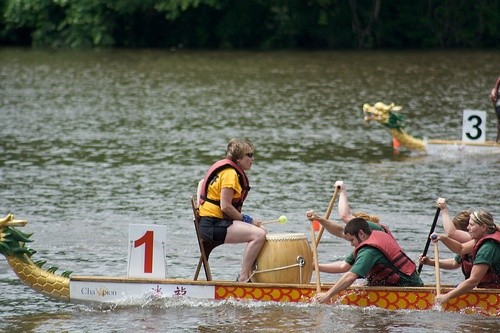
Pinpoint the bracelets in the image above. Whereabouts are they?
[241,213,253,224]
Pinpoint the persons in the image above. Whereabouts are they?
[197,138,267,284]
[306,181,424,304]
[430,210,500,306]
[491,76,500,143]
[419,197,474,280]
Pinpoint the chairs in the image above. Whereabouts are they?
[190,195,222,281]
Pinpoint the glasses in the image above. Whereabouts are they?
[243,152,255,157]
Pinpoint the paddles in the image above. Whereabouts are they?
[417,208,441,273]
[431,235,444,311]
[308,212,322,293]
[312,186,341,253]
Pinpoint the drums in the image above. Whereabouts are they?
[249,232,313,284]
